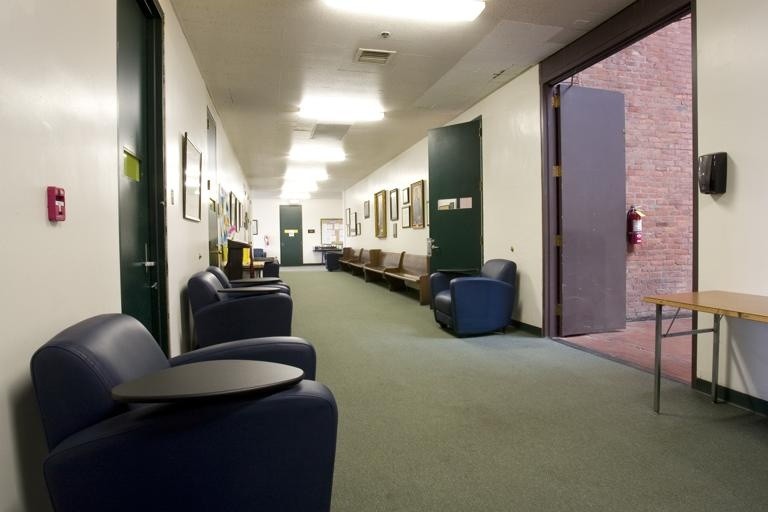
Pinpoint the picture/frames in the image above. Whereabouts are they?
[181,132,259,236]
[320,180,429,245]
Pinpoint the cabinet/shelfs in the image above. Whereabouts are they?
[227,239,254,279]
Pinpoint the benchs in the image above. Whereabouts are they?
[337,247,432,306]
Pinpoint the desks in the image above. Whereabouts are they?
[641,289,768,414]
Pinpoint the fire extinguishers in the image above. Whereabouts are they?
[627,205,646,244]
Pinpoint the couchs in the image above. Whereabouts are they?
[205,265,292,289]
[28,314,339,511]
[187,271,293,348]
[431,258,517,338]
